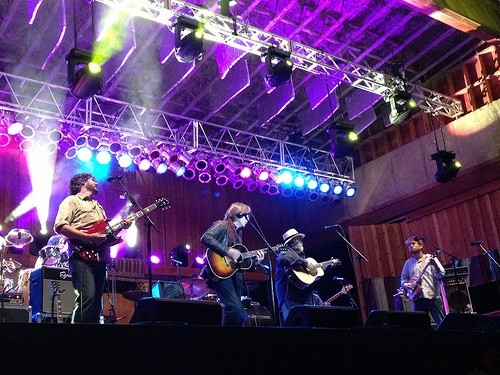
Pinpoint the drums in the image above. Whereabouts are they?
[38,245,61,265]
[18,268,37,305]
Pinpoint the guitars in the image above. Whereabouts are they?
[66,198,172,261]
[203,244,289,282]
[285,257,341,290]
[50,281,63,323]
[403,248,441,301]
[320,283,353,306]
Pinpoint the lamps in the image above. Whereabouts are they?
[427,111,462,184]
[169,244,191,267]
[388,90,422,126]
[10,242,26,255]
[0,109,357,205]
[325,79,358,158]
[170,16,205,64]
[64,0,105,100]
[30,227,52,257]
[260,45,294,88]
[192,255,205,268]
[123,240,140,260]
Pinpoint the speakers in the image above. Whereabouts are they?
[29,265,78,315]
[438,312,500,331]
[362,310,431,330]
[130,298,222,326]
[284,304,364,328]
[444,274,474,313]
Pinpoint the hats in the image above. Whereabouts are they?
[282,228,305,247]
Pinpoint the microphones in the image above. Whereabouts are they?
[333,277,344,281]
[236,212,250,218]
[414,236,426,242]
[324,225,340,231]
[470,240,483,246]
[107,176,124,182]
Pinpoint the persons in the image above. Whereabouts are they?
[53,172,132,324]
[200,202,265,327]
[275,228,338,321]
[399,236,449,326]
